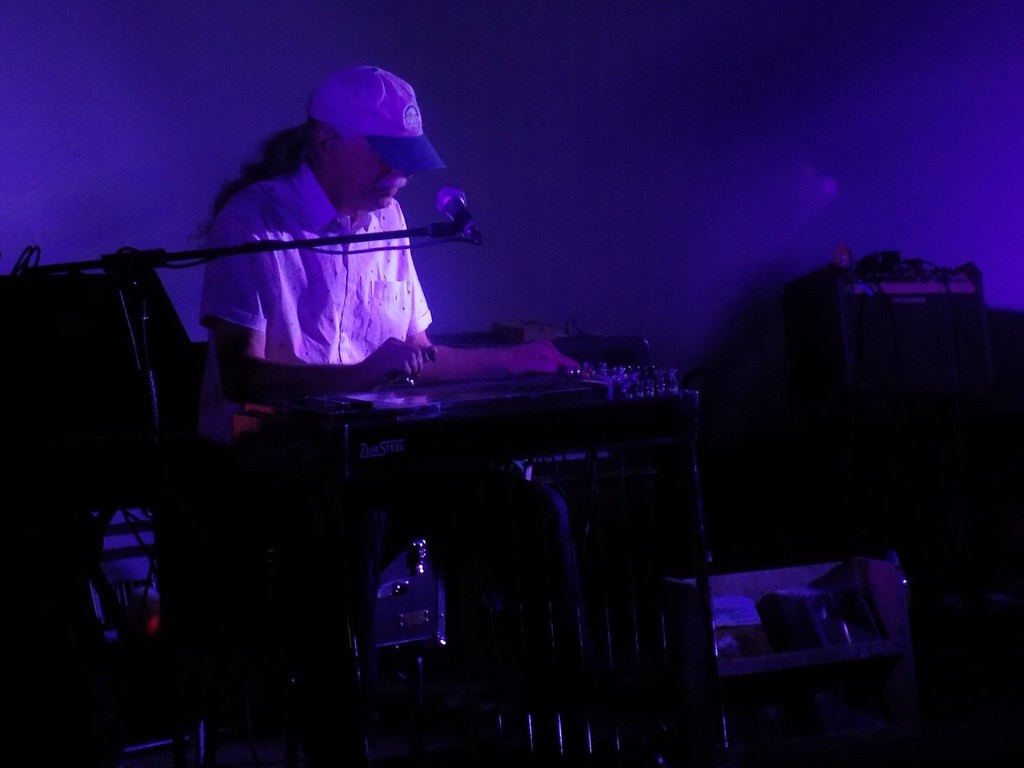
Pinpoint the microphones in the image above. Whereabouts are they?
[436,186,484,244]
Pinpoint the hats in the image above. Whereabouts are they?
[309,66,446,174]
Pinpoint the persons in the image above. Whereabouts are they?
[198,65,585,671]
